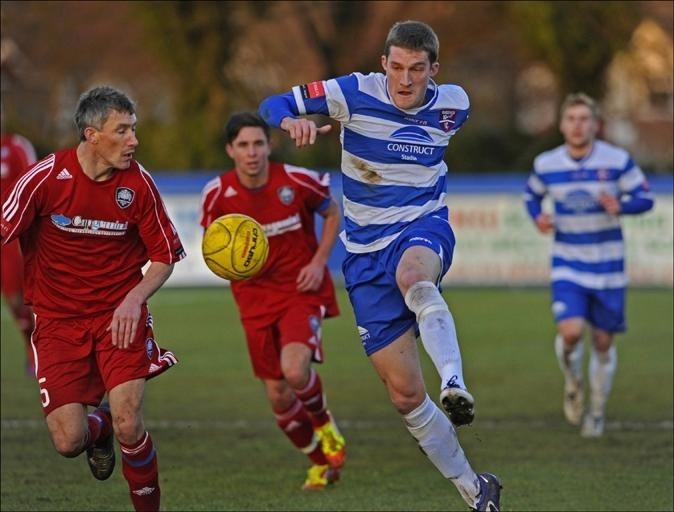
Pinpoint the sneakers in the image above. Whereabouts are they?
[86,403,115,479]
[440,375,475,426]
[468,473,503,511]
[301,463,341,490]
[564,373,605,438]
[307,407,347,468]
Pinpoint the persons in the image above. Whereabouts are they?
[520,93,659,443]
[258,16,502,511]
[0,82,177,512]
[0,130,39,379]
[196,111,350,491]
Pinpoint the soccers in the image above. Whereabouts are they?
[202,214,271,280]
